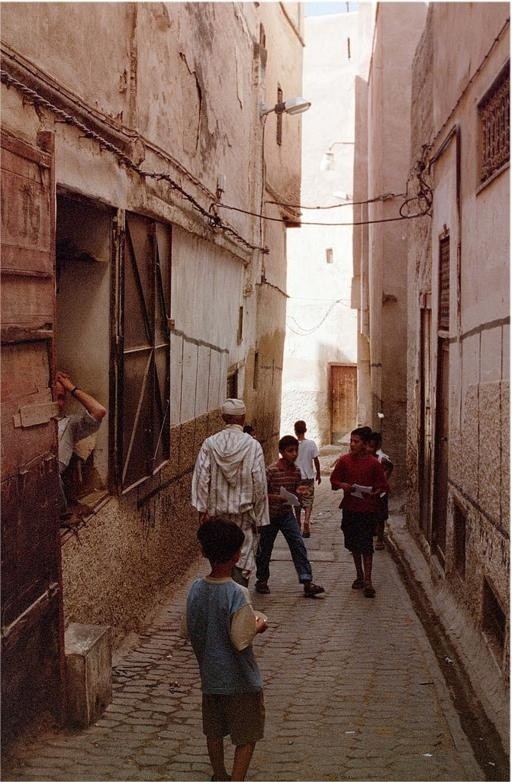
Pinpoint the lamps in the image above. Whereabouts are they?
[260,97,312,118]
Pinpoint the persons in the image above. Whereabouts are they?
[56,371,108,523]
[329,426,393,597]
[244,424,257,440]
[253,436,325,598]
[293,421,321,538]
[189,397,270,590]
[177,517,269,781]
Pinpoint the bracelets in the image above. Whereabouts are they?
[70,386,81,395]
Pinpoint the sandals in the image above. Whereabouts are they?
[352,580,375,598]
[255,581,270,594]
[304,583,324,598]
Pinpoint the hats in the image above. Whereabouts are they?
[221,398,246,416]
